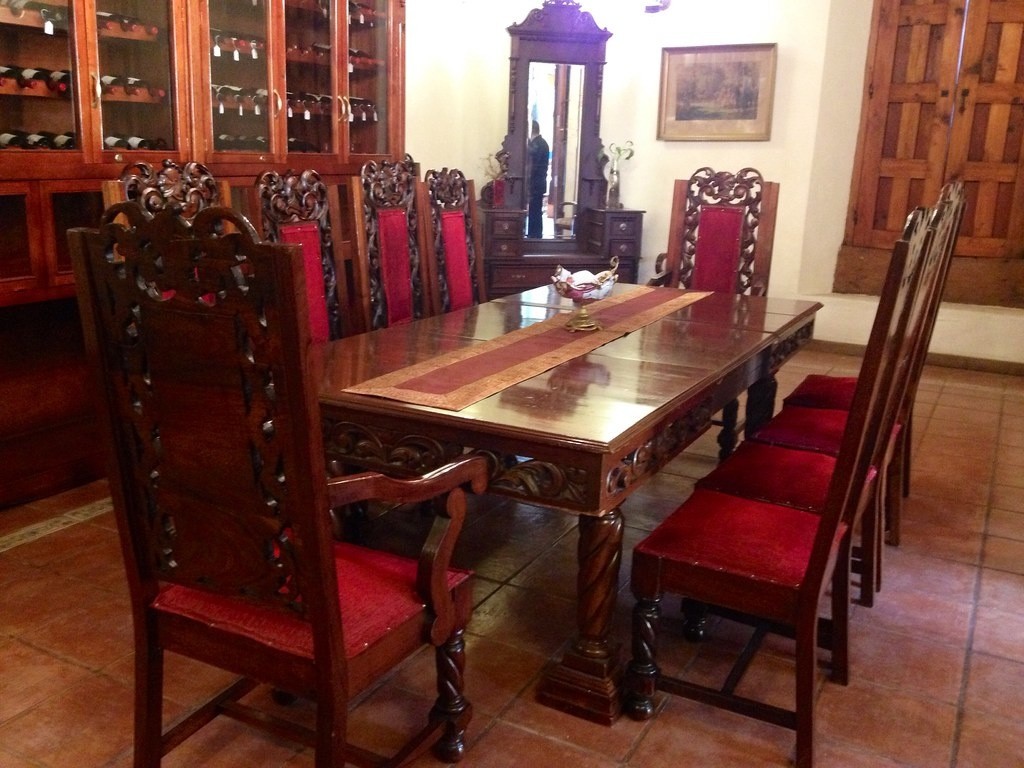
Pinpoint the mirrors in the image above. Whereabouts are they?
[523,59,586,242]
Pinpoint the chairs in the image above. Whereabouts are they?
[65,154,483,768]
[643,168,777,459]
[753,170,966,546]
[695,200,956,607]
[627,201,932,768]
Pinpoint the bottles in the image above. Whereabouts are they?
[317,0,379,19]
[0,130,154,151]
[210,25,386,69]
[0,0,159,36]
[0,62,168,98]
[211,81,379,114]
[215,132,319,153]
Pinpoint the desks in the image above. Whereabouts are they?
[265,283,824,727]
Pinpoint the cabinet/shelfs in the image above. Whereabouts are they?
[476,0,646,303]
[0,0,405,508]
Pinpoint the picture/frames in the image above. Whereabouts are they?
[655,42,777,141]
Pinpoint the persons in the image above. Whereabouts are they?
[527,120,550,239]
